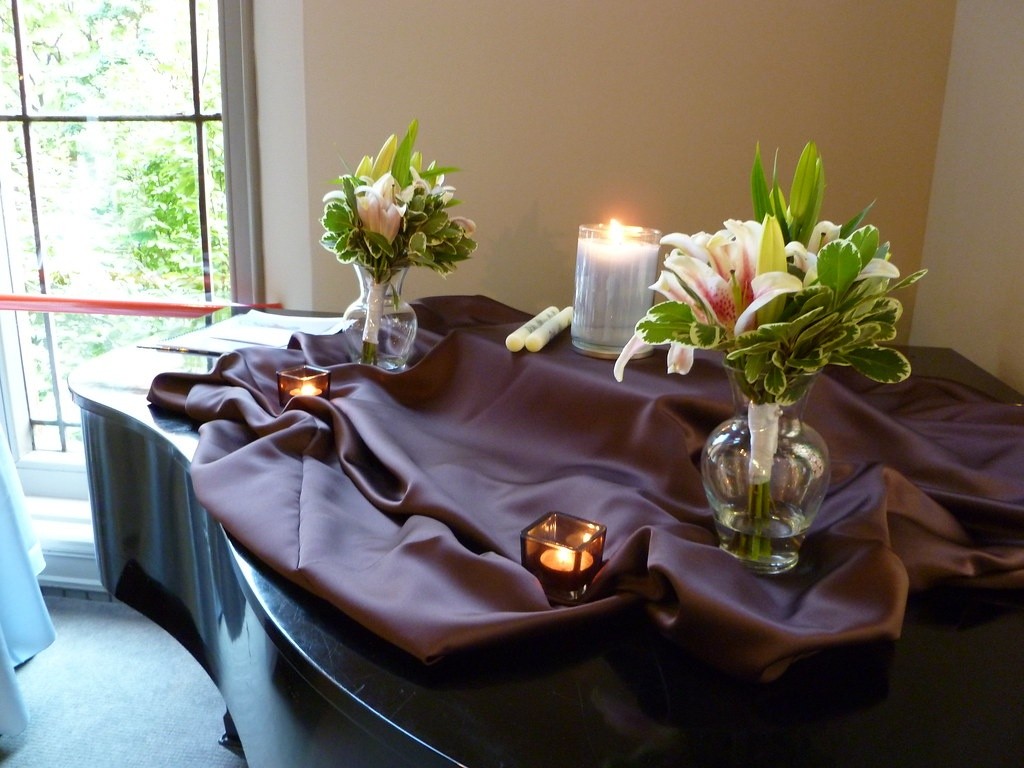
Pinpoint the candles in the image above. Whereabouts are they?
[277,364,331,408]
[572,218,660,356]
[519,512,605,603]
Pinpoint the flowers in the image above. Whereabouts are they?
[617,136,923,402]
[315,115,476,277]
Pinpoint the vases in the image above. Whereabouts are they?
[340,262,417,373]
[701,361,829,580]
